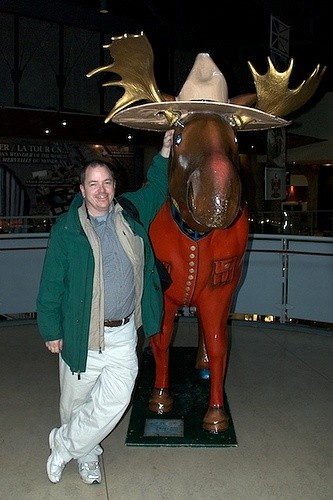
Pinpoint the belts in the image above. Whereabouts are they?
[104,311,133,327]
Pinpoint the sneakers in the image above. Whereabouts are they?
[78,461,102,485]
[46,427,67,483]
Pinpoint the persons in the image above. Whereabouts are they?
[35,129,174,485]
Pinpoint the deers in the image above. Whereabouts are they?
[86,30,327,442]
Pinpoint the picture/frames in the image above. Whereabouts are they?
[265,167,286,201]
